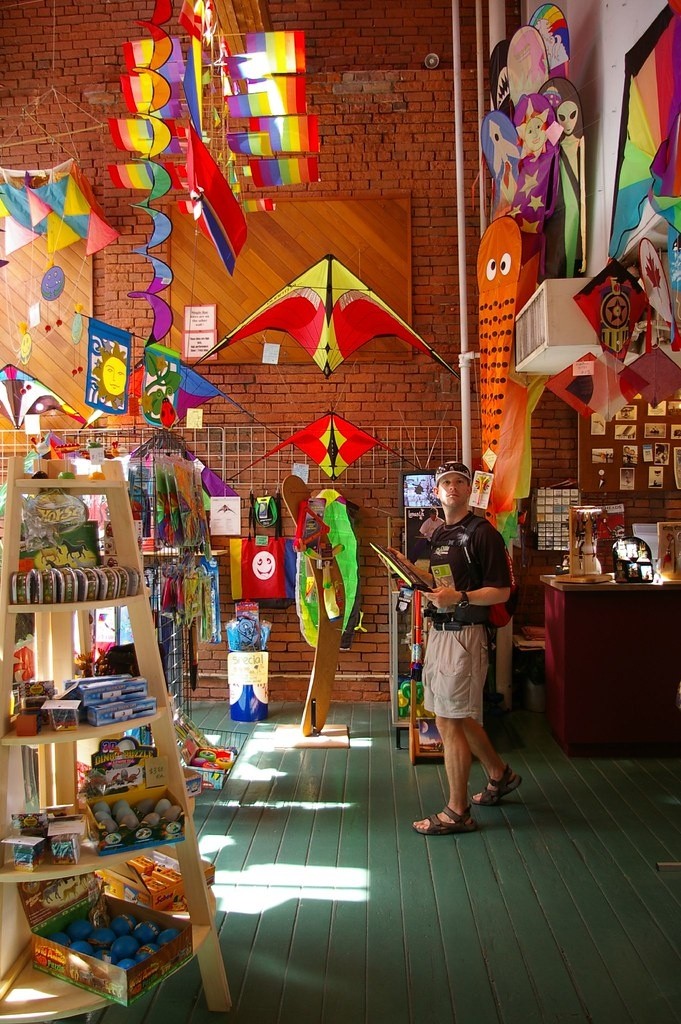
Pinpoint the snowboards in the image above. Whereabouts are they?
[281,474,346,737]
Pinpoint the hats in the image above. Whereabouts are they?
[435,461,472,487]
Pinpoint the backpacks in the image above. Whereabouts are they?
[254,496,277,528]
[430,517,517,627]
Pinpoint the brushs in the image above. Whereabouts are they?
[412,590,423,682]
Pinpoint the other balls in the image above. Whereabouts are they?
[48,913,179,970]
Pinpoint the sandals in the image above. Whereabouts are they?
[412,803,477,834]
[471,763,522,805]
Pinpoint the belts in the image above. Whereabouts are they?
[433,621,481,631]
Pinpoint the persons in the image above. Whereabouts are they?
[417,508,445,558]
[389,461,524,836]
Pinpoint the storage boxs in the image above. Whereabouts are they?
[63,674,132,691]
[17,873,194,1008]
[77,676,149,707]
[85,788,186,855]
[86,695,158,727]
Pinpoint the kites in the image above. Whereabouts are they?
[0,0,681,656]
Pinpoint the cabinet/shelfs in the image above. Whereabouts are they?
[0,458,232,1024]
[410,593,444,765]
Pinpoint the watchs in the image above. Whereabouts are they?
[458,591,469,608]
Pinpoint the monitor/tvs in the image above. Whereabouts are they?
[399,469,442,518]
